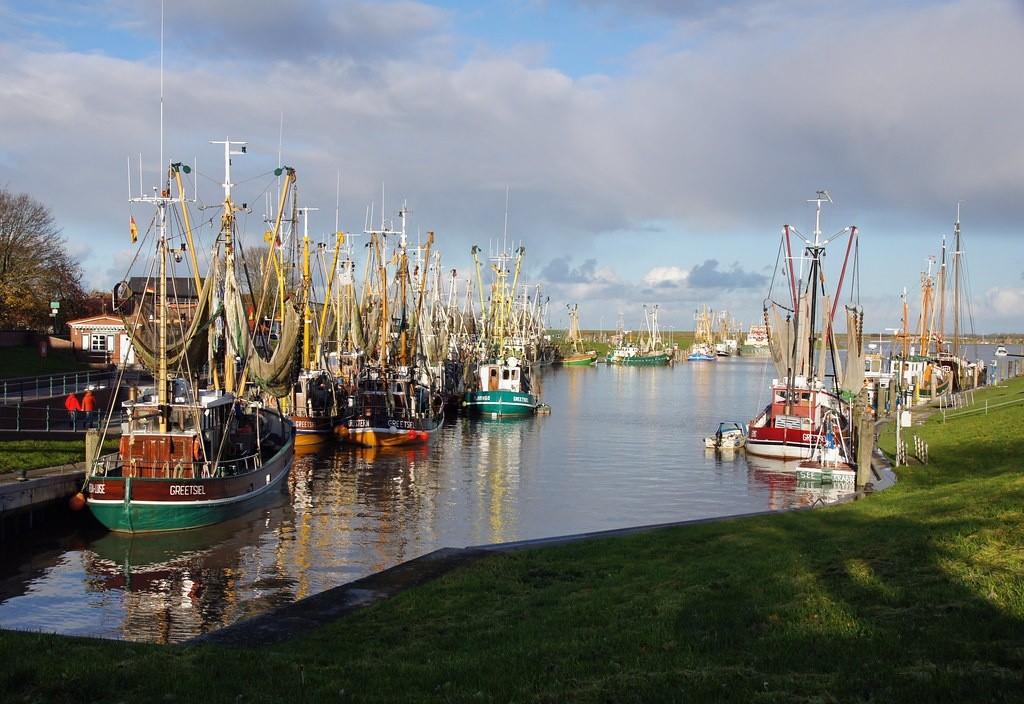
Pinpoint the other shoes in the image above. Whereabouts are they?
[89,426,95,428]
[83,423,86,428]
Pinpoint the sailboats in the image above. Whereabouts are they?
[69,157,1010,536]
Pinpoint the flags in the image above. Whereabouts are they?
[129,215,138,244]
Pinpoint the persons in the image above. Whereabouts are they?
[65,392,82,430]
[82,390,97,428]
